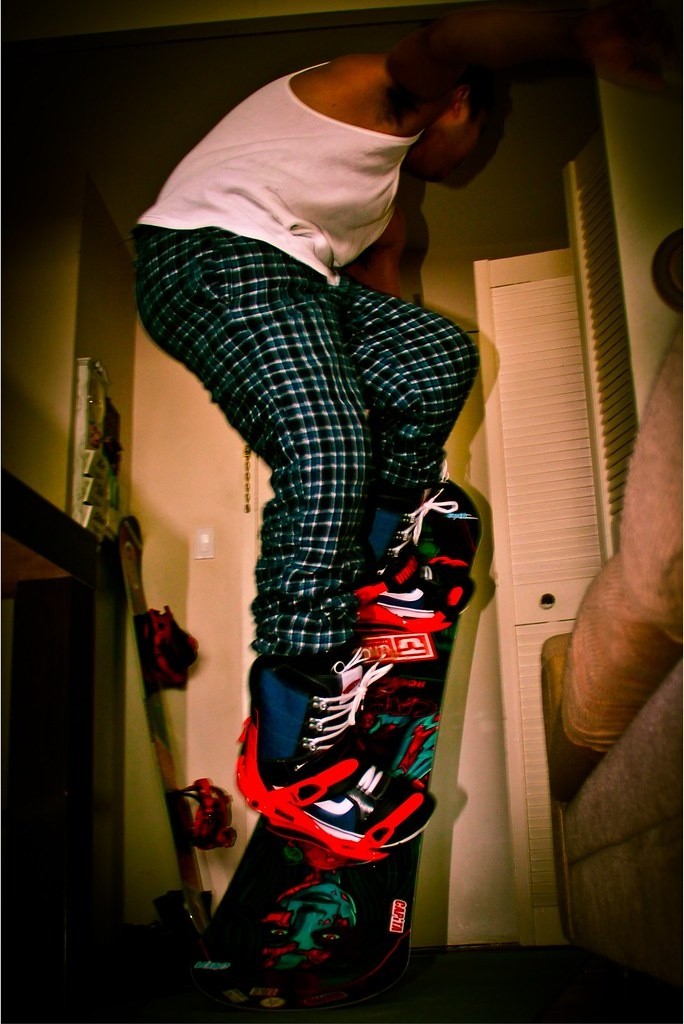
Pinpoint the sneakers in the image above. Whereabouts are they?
[235,759,437,850]
[355,561,476,620]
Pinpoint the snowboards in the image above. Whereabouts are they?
[117,515,237,935]
[193,481,483,1012]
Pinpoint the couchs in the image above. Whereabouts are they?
[541,325,683,987]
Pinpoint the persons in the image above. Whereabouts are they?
[133,0,682,854]
